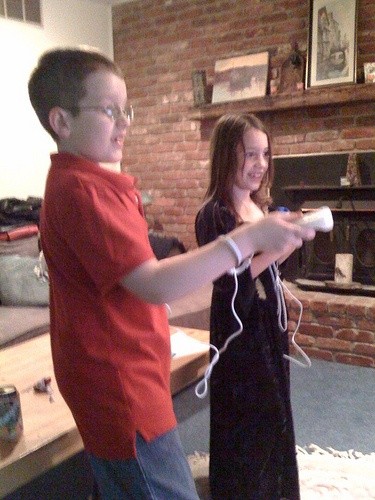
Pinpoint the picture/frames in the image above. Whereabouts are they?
[303,0,361,91]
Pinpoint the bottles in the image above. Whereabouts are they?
[1,384,22,445]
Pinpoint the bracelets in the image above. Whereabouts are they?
[216,232,244,267]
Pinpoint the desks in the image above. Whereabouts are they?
[0,325,209,500]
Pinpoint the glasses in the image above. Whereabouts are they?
[76,103,135,120]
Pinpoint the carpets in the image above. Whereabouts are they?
[185,442,375,500]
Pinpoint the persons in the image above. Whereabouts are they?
[27,47,315,500]
[194,110,304,500]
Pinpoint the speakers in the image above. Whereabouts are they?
[350,214,375,283]
[303,215,349,281]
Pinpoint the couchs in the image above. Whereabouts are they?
[0,224,186,348]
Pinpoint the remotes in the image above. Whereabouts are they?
[290,208,334,237]
